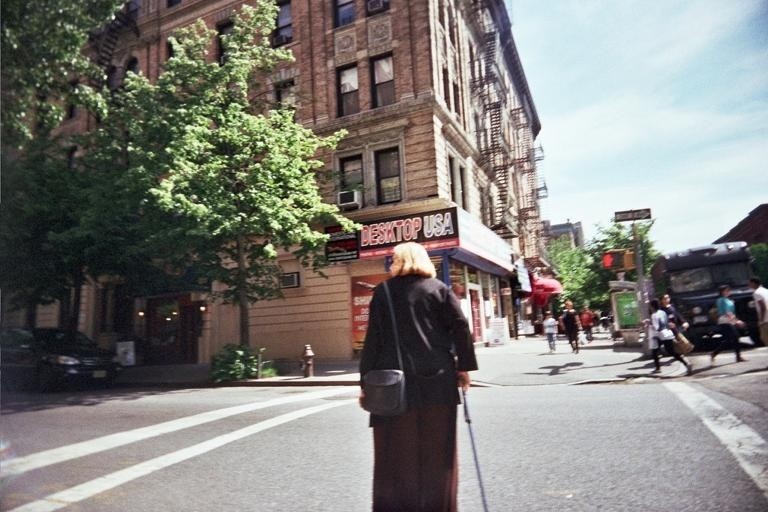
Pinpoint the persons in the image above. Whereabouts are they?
[662,293,689,337]
[559,300,582,353]
[641,298,693,374]
[356,240,480,512]
[581,306,594,339]
[543,310,558,351]
[710,284,747,361]
[746,276,768,346]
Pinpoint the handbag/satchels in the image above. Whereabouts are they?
[362,368,408,416]
[672,331,696,354]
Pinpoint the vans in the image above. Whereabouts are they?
[650,241,763,353]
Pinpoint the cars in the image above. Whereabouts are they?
[0,326,117,393]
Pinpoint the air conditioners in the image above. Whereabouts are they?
[337,190,363,212]
